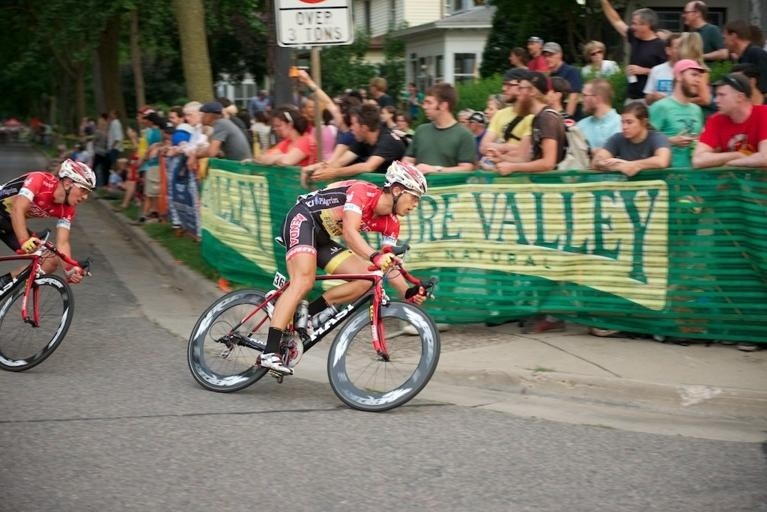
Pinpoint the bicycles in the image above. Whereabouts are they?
[0,225,97,373]
[183,234,444,416]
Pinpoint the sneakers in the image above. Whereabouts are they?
[522,318,567,334]
[31,228,51,246]
[256,350,293,375]
[433,313,454,332]
[0,273,14,295]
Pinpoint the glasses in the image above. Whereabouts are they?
[589,51,601,56]
[579,92,595,99]
[682,10,697,14]
[501,82,518,86]
[517,85,531,92]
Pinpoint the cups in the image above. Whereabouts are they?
[624,74,638,84]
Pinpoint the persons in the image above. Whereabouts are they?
[199,70,479,335]
[592,0,767,351]
[457,38,623,333]
[5,117,53,145]
[57,96,250,239]
[254,159,435,375]
[0,157,96,293]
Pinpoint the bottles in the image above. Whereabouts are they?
[293,299,309,331]
[310,304,339,330]
[687,132,699,158]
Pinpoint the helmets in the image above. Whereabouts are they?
[383,159,427,199]
[58,159,97,192]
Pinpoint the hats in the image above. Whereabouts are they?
[521,72,548,95]
[673,58,711,79]
[540,42,562,56]
[136,106,154,115]
[143,113,158,121]
[711,73,744,93]
[465,113,484,124]
[198,101,223,113]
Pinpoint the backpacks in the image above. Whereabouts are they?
[544,108,590,183]
